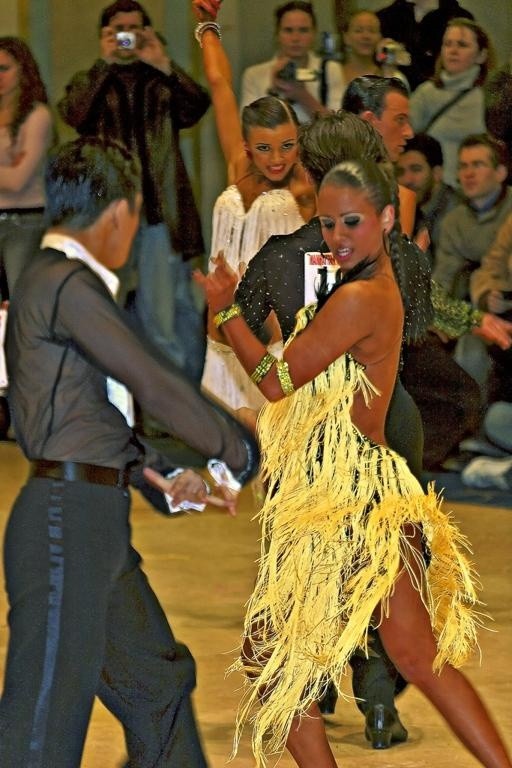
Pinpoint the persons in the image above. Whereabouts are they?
[1,37,54,302]
[336,75,512,471]
[191,2,317,501]
[469,214,512,489]
[194,163,512,768]
[402,19,504,208]
[337,11,412,100]
[393,133,465,273]
[224,112,432,749]
[59,1,209,381]
[239,1,345,132]
[430,136,511,448]
[0,138,254,768]
[374,1,476,98]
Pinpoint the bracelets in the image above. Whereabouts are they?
[212,303,243,330]
[273,360,296,398]
[194,20,224,45]
[248,352,275,388]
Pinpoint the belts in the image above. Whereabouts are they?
[31,457,130,487]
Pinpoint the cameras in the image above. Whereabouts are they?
[109,29,139,53]
[273,62,318,85]
[375,41,408,67]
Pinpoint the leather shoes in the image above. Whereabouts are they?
[365,705,407,750]
[318,680,339,714]
